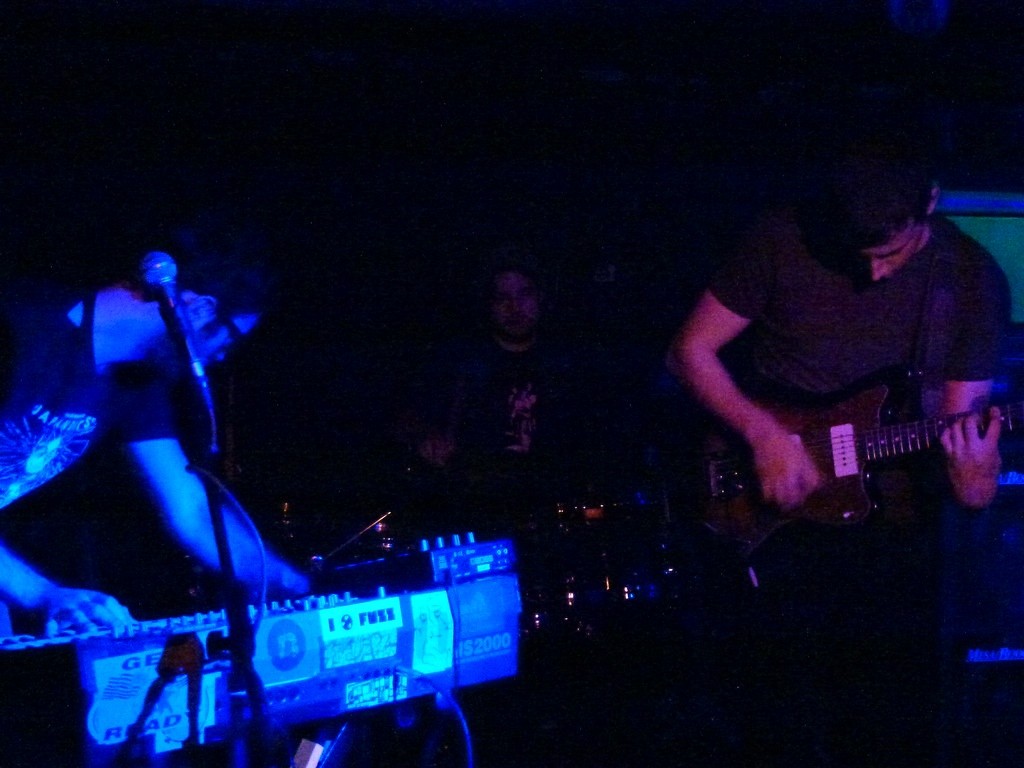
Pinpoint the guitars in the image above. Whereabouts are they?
[701,385,1024,527]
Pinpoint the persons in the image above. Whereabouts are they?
[390,231,637,768]
[660,104,1015,768]
[0,196,312,766]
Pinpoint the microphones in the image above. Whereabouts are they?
[141,251,215,423]
